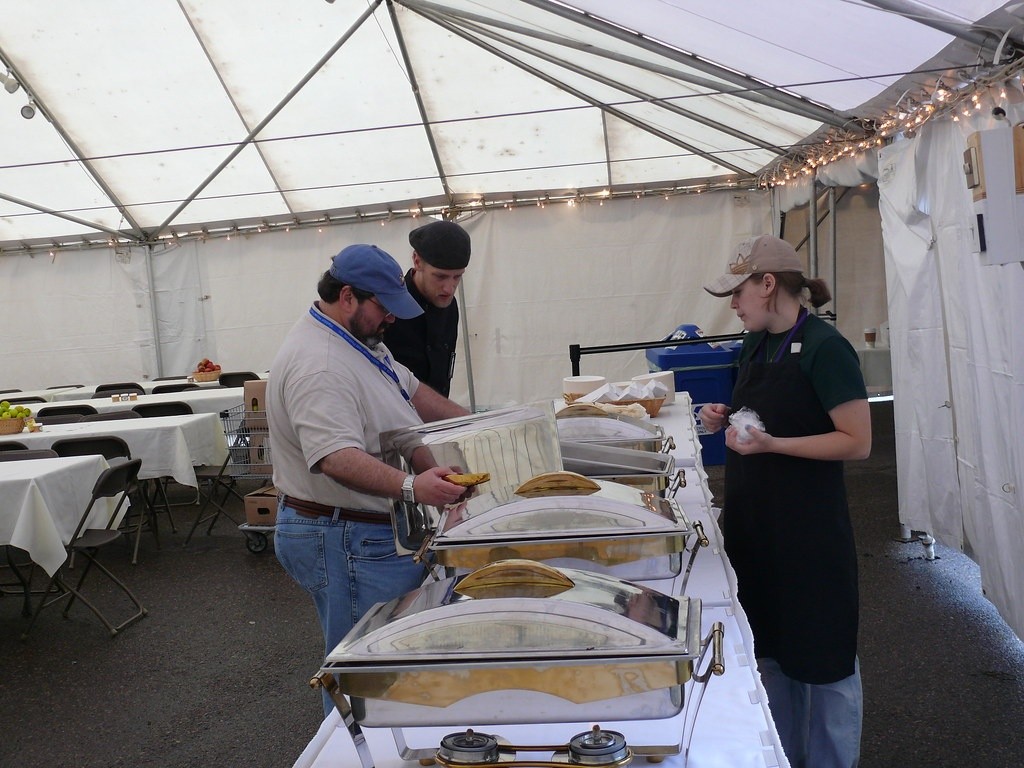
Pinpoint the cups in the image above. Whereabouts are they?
[864,328,876,348]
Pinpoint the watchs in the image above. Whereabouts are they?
[400,474,417,507]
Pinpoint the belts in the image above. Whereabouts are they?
[277,493,391,525]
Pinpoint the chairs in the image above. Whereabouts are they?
[20,458,149,643]
[133,401,220,509]
[0,442,28,451]
[52,435,160,566]
[34,414,84,425]
[92,389,144,399]
[77,410,143,423]
[219,372,260,387]
[182,419,250,546]
[96,382,146,395]
[152,384,200,394]
[0,397,48,403]
[37,405,98,416]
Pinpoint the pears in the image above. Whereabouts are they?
[0,401,30,418]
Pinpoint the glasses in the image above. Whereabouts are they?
[350,289,391,318]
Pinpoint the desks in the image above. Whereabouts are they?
[0,413,233,547]
[291,391,792,768]
[0,454,104,618]
[10,386,248,458]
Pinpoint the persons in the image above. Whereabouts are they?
[381,221,472,399]
[697,233,873,768]
[265,244,474,719]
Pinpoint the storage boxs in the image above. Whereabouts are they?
[111,394,120,402]
[431,498,692,582]
[250,428,273,474]
[589,426,667,452]
[244,380,268,430]
[121,394,128,401]
[129,393,137,400]
[244,486,278,526]
[323,597,703,728]
[589,458,676,498]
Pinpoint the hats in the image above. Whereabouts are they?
[704,233,804,297]
[409,221,471,270]
[328,244,425,320]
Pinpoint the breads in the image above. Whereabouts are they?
[444,470,490,485]
